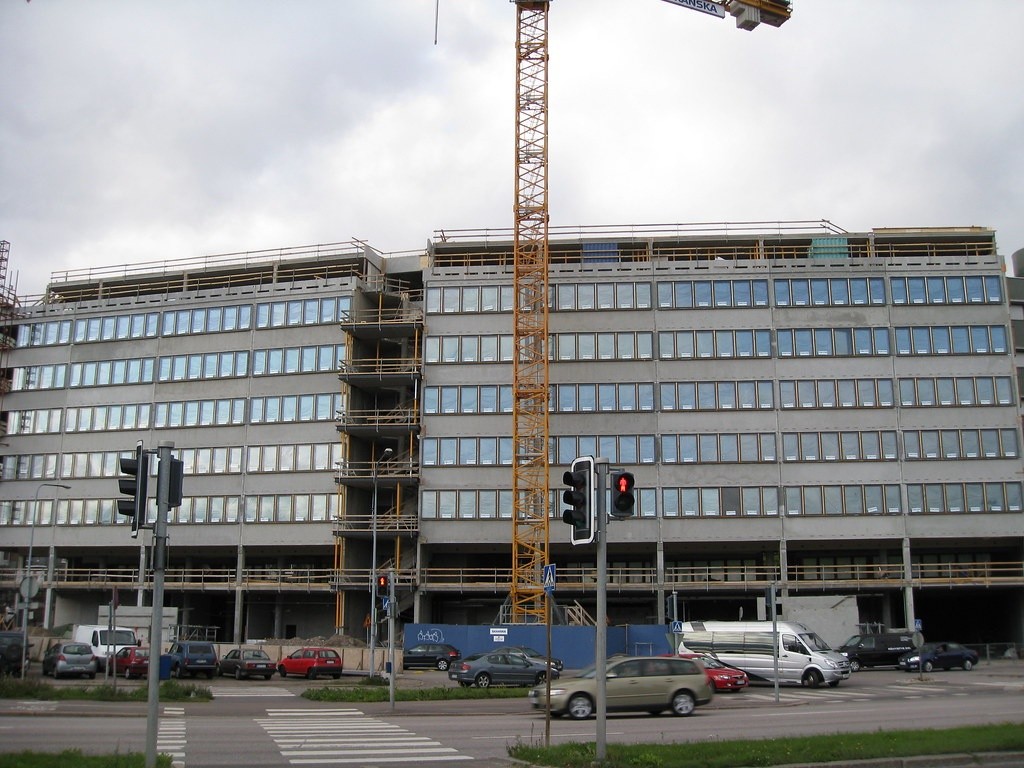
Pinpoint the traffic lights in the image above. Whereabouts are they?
[377,574,388,598]
[117,440,148,539]
[610,472,635,518]
[562,455,595,545]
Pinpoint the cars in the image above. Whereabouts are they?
[657,654,750,692]
[528,656,714,720]
[898,642,979,672]
[42,642,98,679]
[448,647,564,688]
[278,648,342,680]
[220,649,276,680]
[404,643,461,671]
[108,647,151,679]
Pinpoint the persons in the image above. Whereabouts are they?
[618,665,633,676]
[937,646,945,653]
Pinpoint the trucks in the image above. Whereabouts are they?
[75,625,141,673]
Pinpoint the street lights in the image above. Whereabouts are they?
[370,448,393,674]
[22,484,72,681]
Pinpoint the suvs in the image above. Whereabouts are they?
[165,642,219,679]
[0,632,35,677]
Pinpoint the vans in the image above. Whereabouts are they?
[839,634,918,672]
[678,621,851,688]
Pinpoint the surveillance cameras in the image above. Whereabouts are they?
[385,448,393,456]
